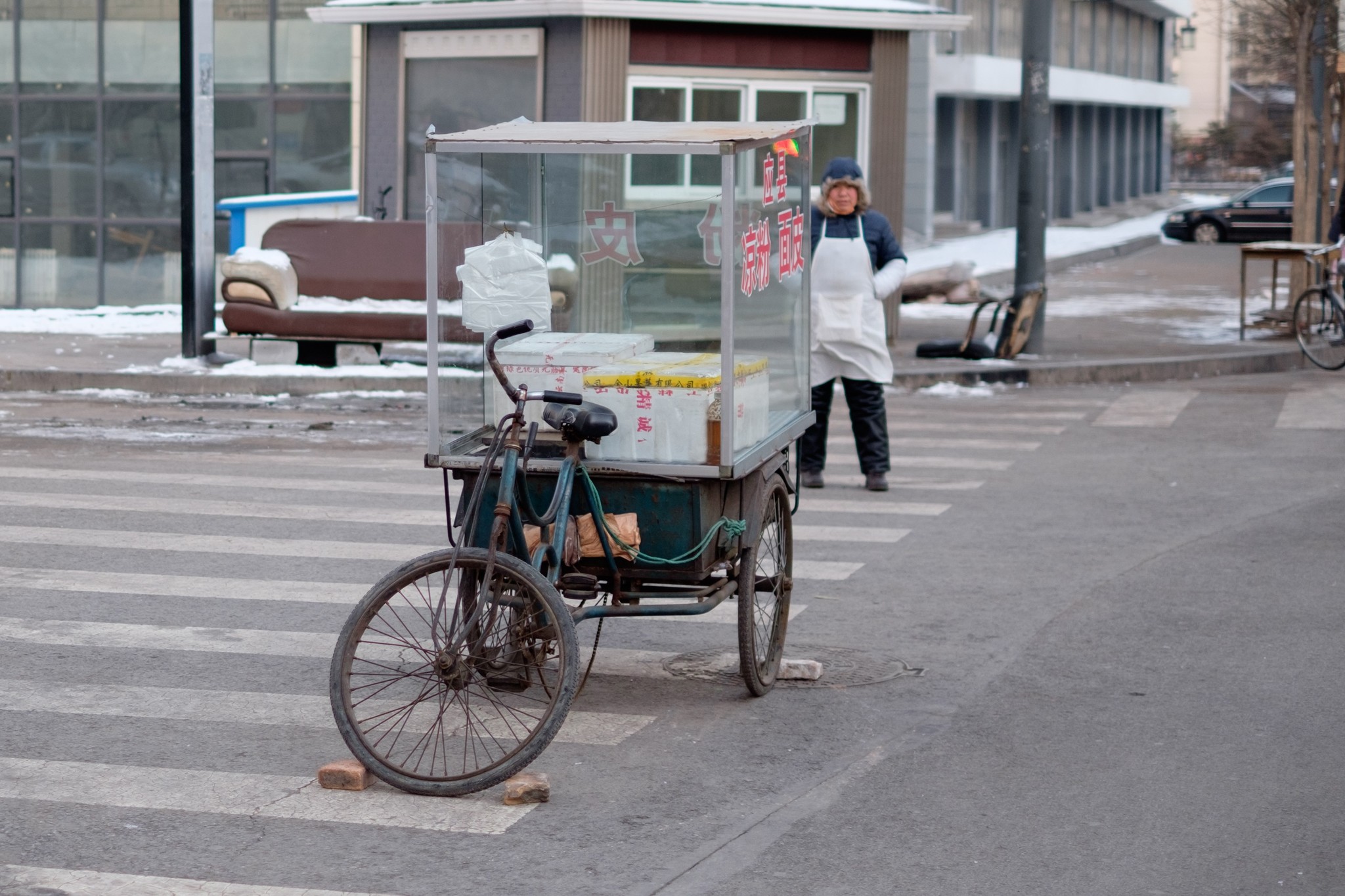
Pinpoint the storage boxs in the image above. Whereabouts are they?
[493,331,655,431]
[579,352,769,466]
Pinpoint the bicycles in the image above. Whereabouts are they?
[1291,235,1345,371]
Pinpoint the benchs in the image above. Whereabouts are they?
[221,217,579,368]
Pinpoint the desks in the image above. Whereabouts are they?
[1239,242,1335,343]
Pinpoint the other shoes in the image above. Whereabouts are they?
[800,471,824,488]
[865,472,889,492]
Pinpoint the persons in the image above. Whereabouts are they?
[796,156,908,490]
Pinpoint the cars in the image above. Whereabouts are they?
[1159,175,1345,245]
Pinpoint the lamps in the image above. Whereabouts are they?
[1180,18,1196,50]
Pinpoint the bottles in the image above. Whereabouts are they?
[706,391,721,467]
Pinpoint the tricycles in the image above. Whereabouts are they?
[327,317,795,799]
